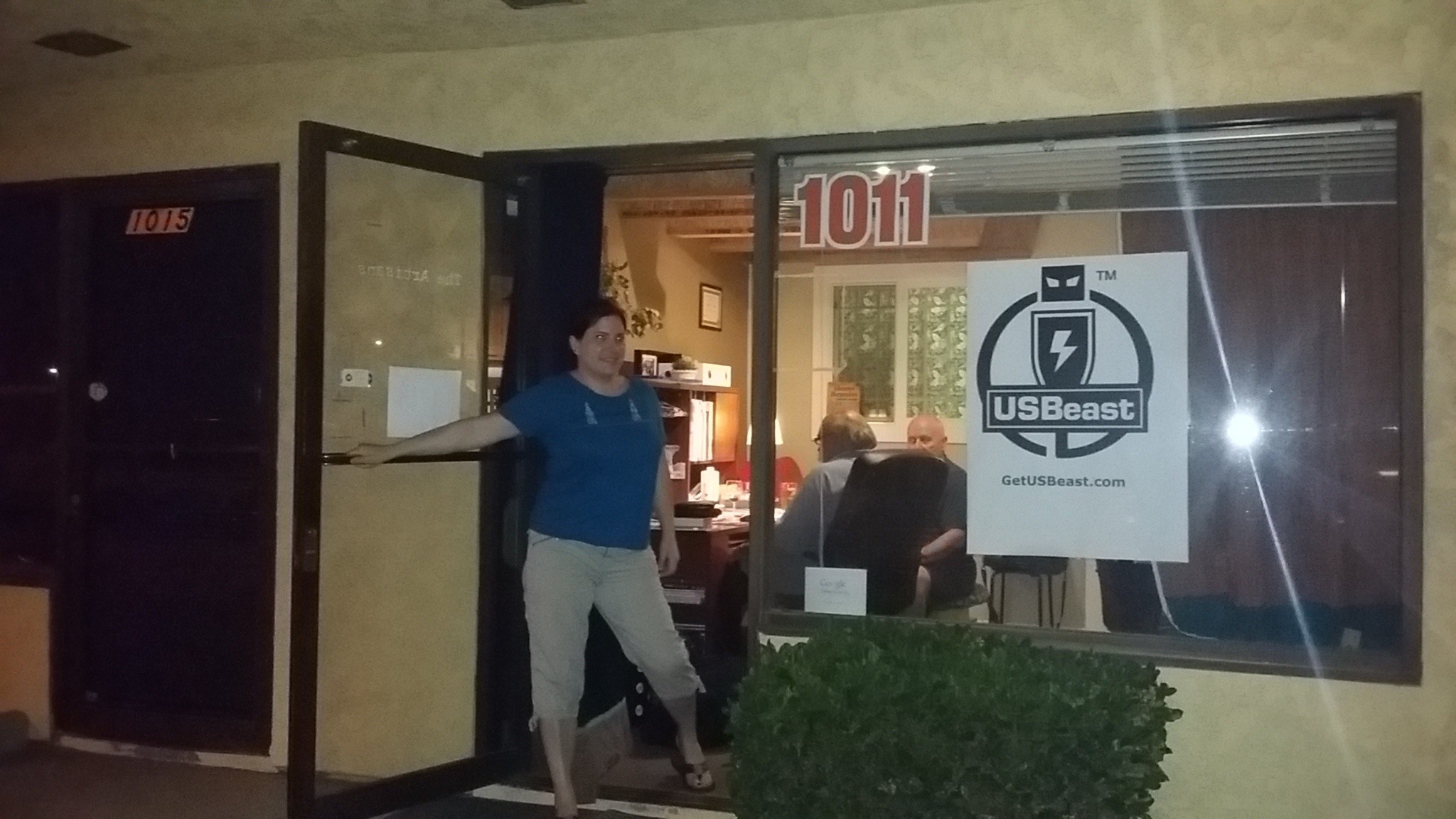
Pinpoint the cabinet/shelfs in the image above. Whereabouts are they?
[638,378,739,505]
[644,507,795,749]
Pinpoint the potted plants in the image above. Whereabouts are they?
[672,357,698,381]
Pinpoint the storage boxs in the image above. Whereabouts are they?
[697,363,732,388]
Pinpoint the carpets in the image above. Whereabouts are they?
[386,796,662,819]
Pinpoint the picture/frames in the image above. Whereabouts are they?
[700,282,724,332]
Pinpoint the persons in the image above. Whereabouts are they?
[906,415,977,618]
[703,410,878,747]
[344,294,715,819]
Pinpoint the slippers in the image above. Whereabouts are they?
[671,748,715,792]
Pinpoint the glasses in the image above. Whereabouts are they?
[814,434,828,445]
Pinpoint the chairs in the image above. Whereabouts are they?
[736,456,804,499]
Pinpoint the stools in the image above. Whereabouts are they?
[981,556,1068,628]
[925,582,990,621]
